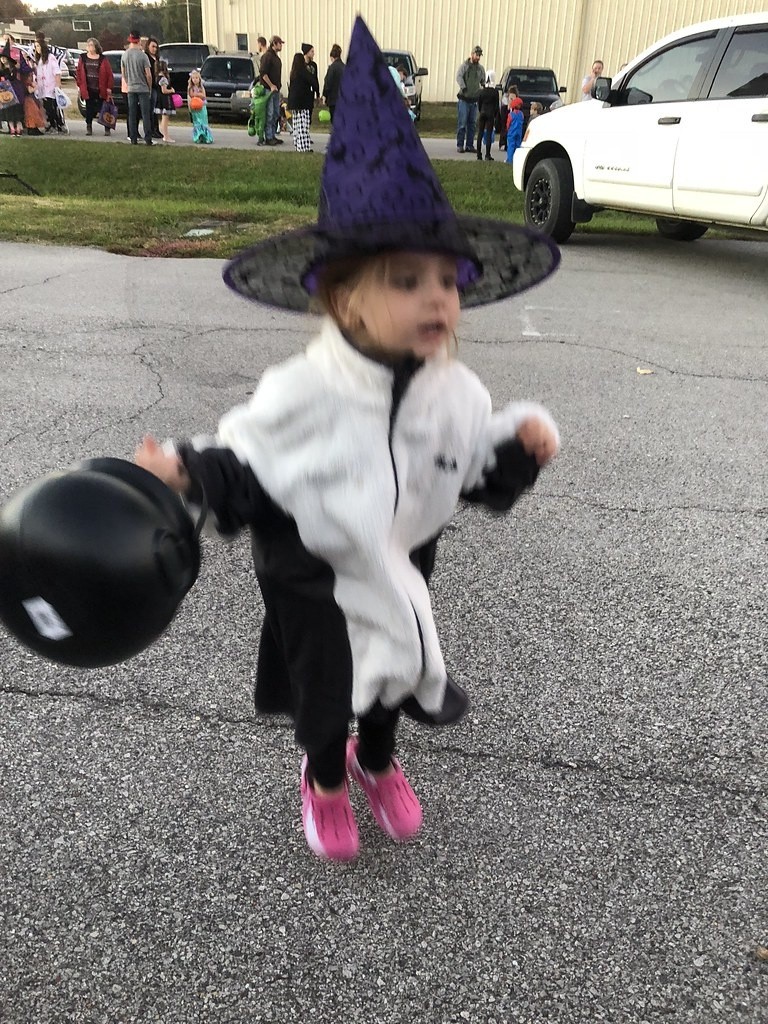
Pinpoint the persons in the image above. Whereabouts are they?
[0,26,69,137]
[497,85,542,163]
[456,46,486,154]
[76,37,115,136]
[288,43,320,153]
[187,69,213,144]
[323,44,346,124]
[582,60,604,101]
[250,36,284,153]
[476,70,501,160]
[134,247,562,863]
[119,32,176,143]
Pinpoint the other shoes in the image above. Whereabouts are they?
[163,138,176,144]
[485,155,495,161]
[274,138,283,144]
[476,153,482,160]
[465,146,477,152]
[266,137,276,146]
[85,125,92,135]
[104,126,110,136]
[457,146,464,153]
[28,128,43,136]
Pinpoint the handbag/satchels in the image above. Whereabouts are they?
[98,98,118,130]
[0,79,20,109]
[56,87,72,108]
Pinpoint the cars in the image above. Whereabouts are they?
[0,40,85,82]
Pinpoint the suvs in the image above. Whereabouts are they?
[198,51,262,119]
[156,42,217,101]
[379,48,429,123]
[495,65,568,134]
[75,49,140,118]
[511,10,768,246]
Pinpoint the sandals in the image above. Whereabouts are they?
[348,740,424,839]
[301,754,359,860]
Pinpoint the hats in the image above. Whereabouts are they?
[0,38,17,65]
[269,35,285,43]
[16,51,33,73]
[127,30,140,42]
[301,43,313,53]
[472,46,483,56]
[330,44,342,57]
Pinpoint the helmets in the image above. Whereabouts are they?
[223,16,562,315]
